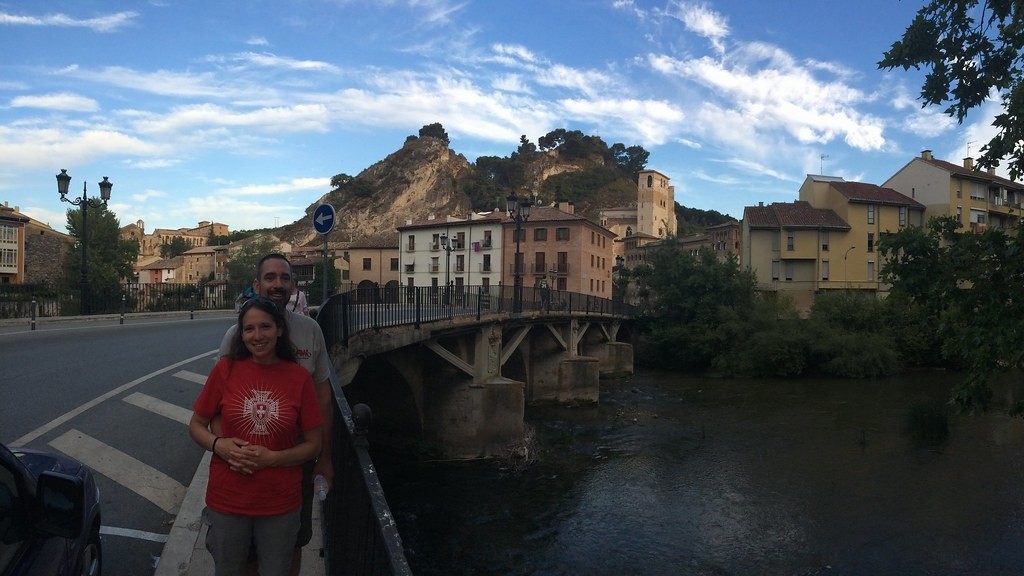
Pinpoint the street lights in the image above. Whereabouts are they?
[504,191,532,314]
[55,168,113,316]
[439,232,458,305]
[615,255,625,315]
[548,268,558,308]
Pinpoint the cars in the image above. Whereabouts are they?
[0,440,105,576]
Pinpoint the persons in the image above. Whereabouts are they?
[241,286,259,301]
[286,273,310,317]
[538,275,551,310]
[189,299,323,576]
[222,254,333,576]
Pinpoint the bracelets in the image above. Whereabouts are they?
[212,437,220,455]
